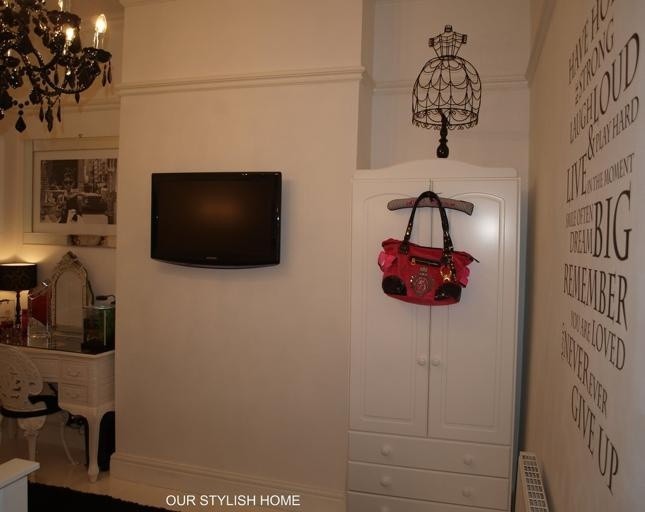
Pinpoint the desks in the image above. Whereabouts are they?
[0,337,115,483]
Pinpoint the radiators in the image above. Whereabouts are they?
[515,452,550,512]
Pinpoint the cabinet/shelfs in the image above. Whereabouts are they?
[352,159,523,512]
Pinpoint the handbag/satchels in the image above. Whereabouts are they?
[379,237,480,305]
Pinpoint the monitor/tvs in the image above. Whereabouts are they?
[148,170,283,271]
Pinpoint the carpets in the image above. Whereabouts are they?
[27,482,181,512]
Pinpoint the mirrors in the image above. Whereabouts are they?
[51,251,94,338]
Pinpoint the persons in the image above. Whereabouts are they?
[56,170,89,226]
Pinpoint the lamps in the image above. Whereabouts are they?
[0,0,113,131]
[0,262,38,328]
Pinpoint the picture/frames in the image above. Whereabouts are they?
[22,136,118,250]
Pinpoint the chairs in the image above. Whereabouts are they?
[0,347,77,484]
